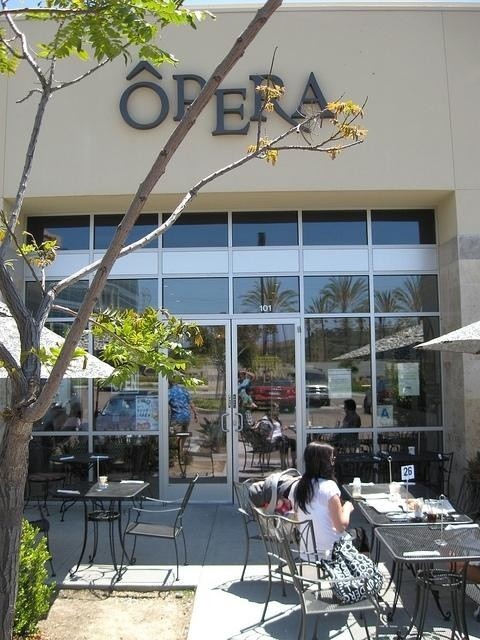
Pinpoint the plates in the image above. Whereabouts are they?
[386,511,427,522]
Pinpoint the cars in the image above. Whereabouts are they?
[99,383,120,393]
[362,373,398,416]
[247,374,297,411]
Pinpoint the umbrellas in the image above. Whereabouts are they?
[414,320,479,356]
[0,300,122,382]
[327,327,422,366]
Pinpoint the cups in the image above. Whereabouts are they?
[425,498,439,520]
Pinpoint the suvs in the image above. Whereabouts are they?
[71,391,181,478]
[288,365,333,407]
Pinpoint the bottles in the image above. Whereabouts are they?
[353,476,362,497]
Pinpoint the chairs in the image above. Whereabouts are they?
[233,480,296,583]
[22,413,220,580]
[276,525,392,640]
[241,409,427,477]
[248,504,337,626]
[233,443,480,521]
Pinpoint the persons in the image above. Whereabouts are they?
[447,537,480,623]
[166,372,200,465]
[233,367,297,470]
[283,441,374,565]
[34,396,85,456]
[330,397,363,448]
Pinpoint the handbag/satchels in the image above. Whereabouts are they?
[315,536,384,606]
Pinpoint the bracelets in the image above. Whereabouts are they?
[194,412,198,416]
